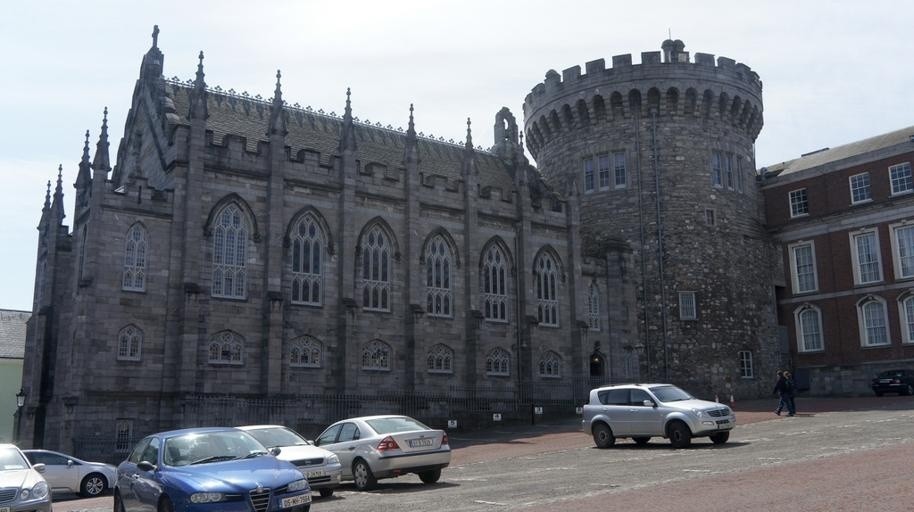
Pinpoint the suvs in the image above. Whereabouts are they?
[579,381,734,451]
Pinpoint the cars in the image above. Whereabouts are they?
[871,366,913,394]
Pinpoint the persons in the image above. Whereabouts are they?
[783,372,796,415]
[773,370,794,416]
